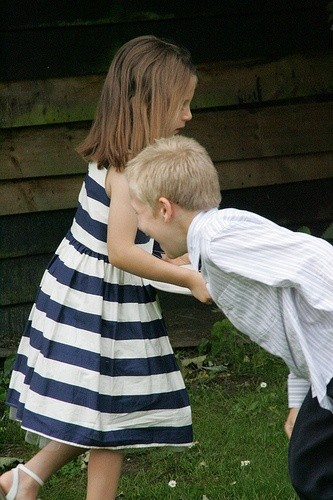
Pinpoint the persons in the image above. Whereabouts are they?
[0,39,215,499]
[127,137,331,499]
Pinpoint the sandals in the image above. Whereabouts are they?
[0,464,43,500]
